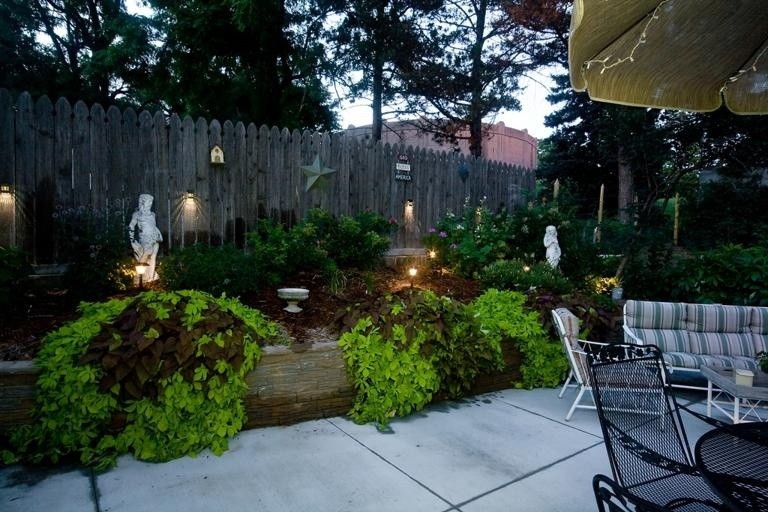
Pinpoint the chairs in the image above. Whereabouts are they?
[551,309,673,432]
[586,342,768,512]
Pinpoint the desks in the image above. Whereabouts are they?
[694,421,768,511]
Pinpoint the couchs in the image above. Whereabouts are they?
[623,300,768,391]
